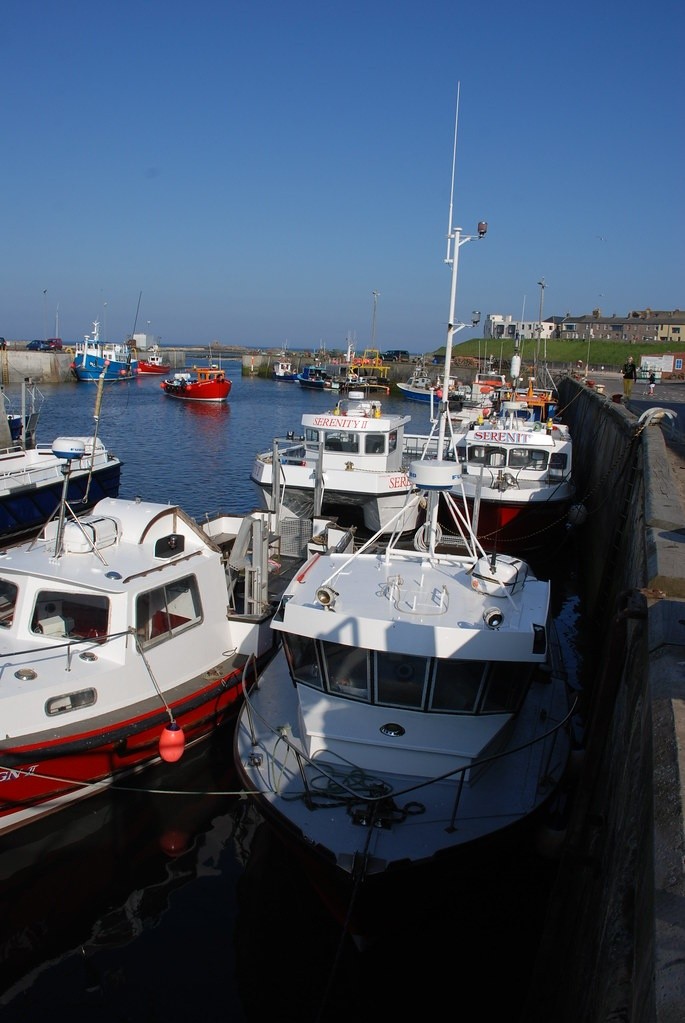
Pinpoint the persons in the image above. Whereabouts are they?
[622,356,637,403]
[647,369,656,395]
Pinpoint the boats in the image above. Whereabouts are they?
[0,439,357,836]
[71,322,139,381]
[399,361,471,406]
[442,371,575,552]
[297,361,340,389]
[233,82,580,896]
[162,364,232,402]
[273,357,300,384]
[0,373,124,553]
[138,356,170,376]
[250,388,451,550]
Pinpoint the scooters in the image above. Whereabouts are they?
[642,378,657,396]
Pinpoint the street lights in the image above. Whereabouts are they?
[537,282,547,362]
[372,291,381,346]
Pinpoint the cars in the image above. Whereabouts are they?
[0,337,6,350]
[26,340,57,351]
[380,349,409,362]
[47,338,62,351]
[353,349,382,366]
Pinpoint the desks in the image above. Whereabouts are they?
[247,535,282,563]
[210,532,237,559]
